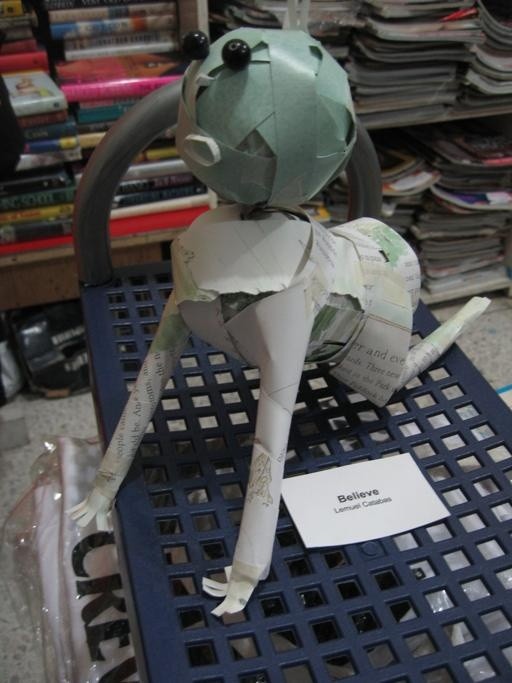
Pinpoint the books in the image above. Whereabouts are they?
[213,1,511,110]
[308,122,512,293]
[1,0,210,258]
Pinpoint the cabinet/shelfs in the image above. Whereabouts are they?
[178,0,511,305]
[1,224,182,309]
